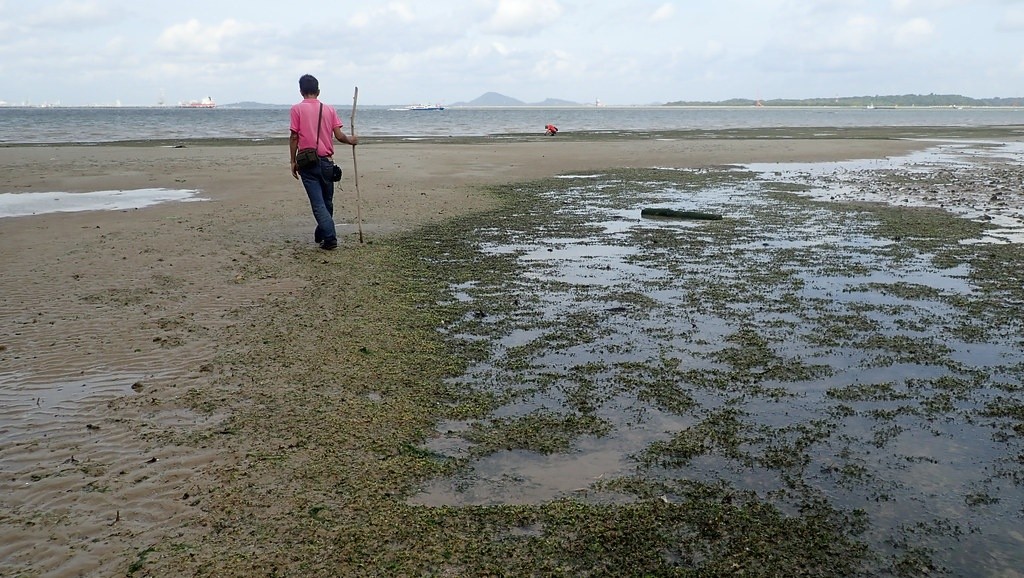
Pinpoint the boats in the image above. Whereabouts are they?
[406,105,444,111]
[175,96,216,108]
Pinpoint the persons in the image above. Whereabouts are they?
[545,123,557,136]
[290,73,358,250]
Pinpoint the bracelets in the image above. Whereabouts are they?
[291,160,295,163]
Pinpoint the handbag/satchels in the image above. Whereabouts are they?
[333,165,341,182]
[554,127,558,131]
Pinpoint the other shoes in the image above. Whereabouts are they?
[315,237,337,249]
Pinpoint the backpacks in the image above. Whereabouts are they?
[295,148,320,166]
